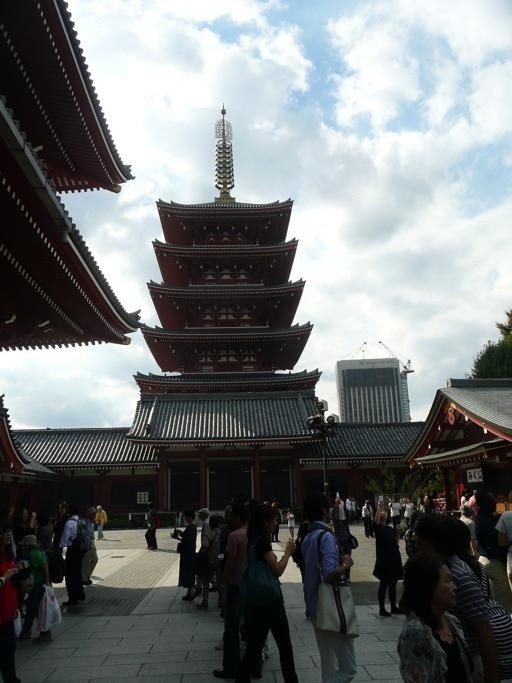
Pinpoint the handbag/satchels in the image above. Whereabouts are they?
[314,582,359,637]
[241,560,282,604]
[38,587,61,631]
[191,553,209,573]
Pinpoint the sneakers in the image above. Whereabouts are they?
[183,587,219,610]
[63,599,78,607]
[391,607,403,614]
[212,642,270,678]
[379,609,391,616]
[82,578,93,585]
[19,632,51,644]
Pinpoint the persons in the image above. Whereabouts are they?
[1,493,109,683]
[362,495,512,682]
[143,499,358,682]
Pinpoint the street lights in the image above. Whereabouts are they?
[304,396,341,493]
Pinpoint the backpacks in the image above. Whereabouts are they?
[69,517,90,552]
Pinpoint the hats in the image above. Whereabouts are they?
[87,507,96,515]
[18,535,36,546]
